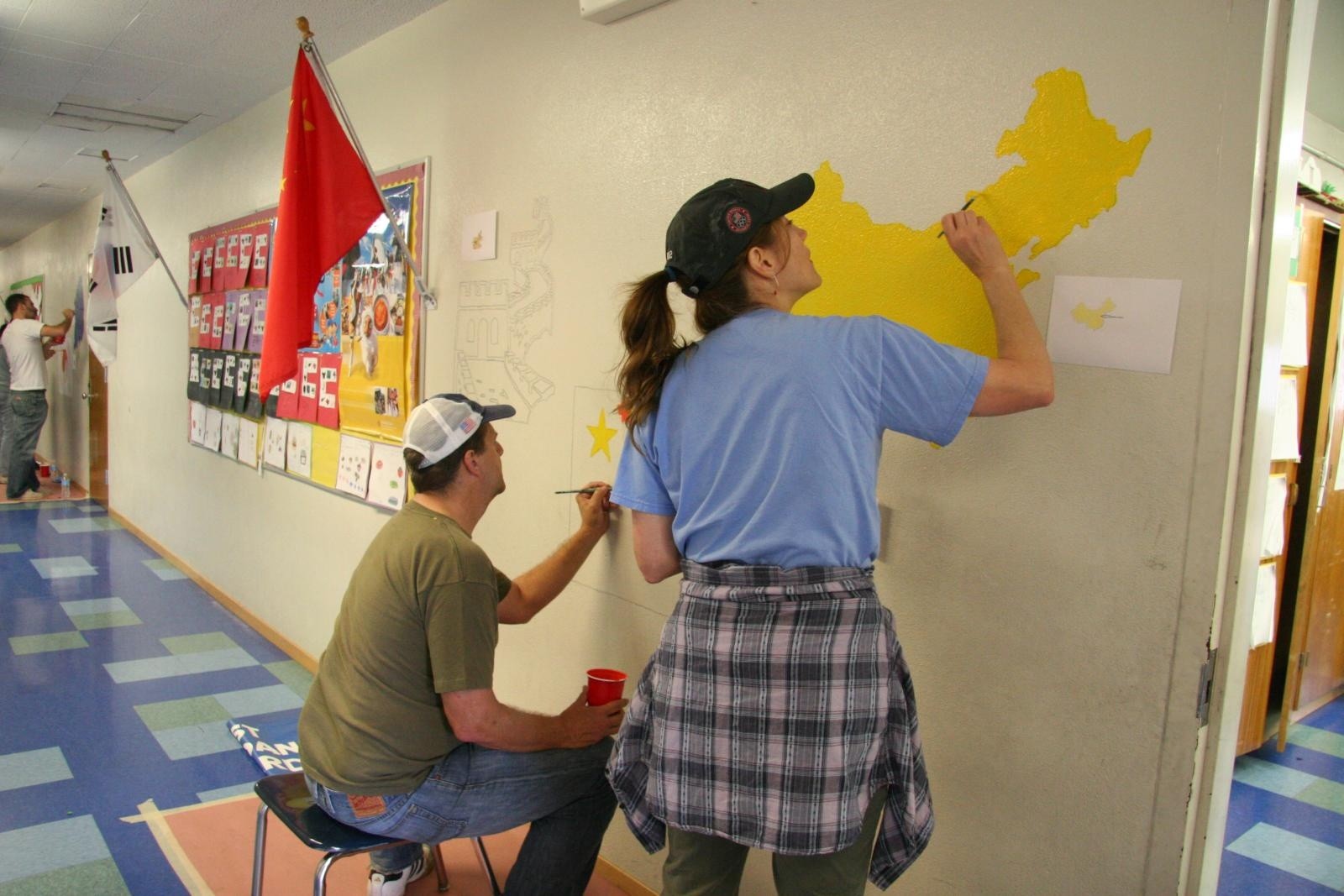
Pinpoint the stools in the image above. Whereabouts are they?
[251,770,500,896]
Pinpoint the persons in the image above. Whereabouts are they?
[605,174,1057,896]
[0,293,76,501]
[301,394,629,896]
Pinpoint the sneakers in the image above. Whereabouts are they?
[366,846,434,896]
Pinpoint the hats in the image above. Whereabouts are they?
[667,173,814,297]
[403,393,516,470]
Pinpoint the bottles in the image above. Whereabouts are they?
[51,462,59,482]
[62,473,71,497]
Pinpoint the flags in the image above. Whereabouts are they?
[257,43,383,404]
[86,163,159,366]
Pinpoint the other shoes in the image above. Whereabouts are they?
[20,488,43,500]
[34,459,41,469]
[0,474,9,483]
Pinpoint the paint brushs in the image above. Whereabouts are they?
[555,488,611,493]
[937,196,976,238]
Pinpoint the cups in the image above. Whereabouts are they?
[586,669,627,707]
[41,466,49,477]
[53,335,65,343]
